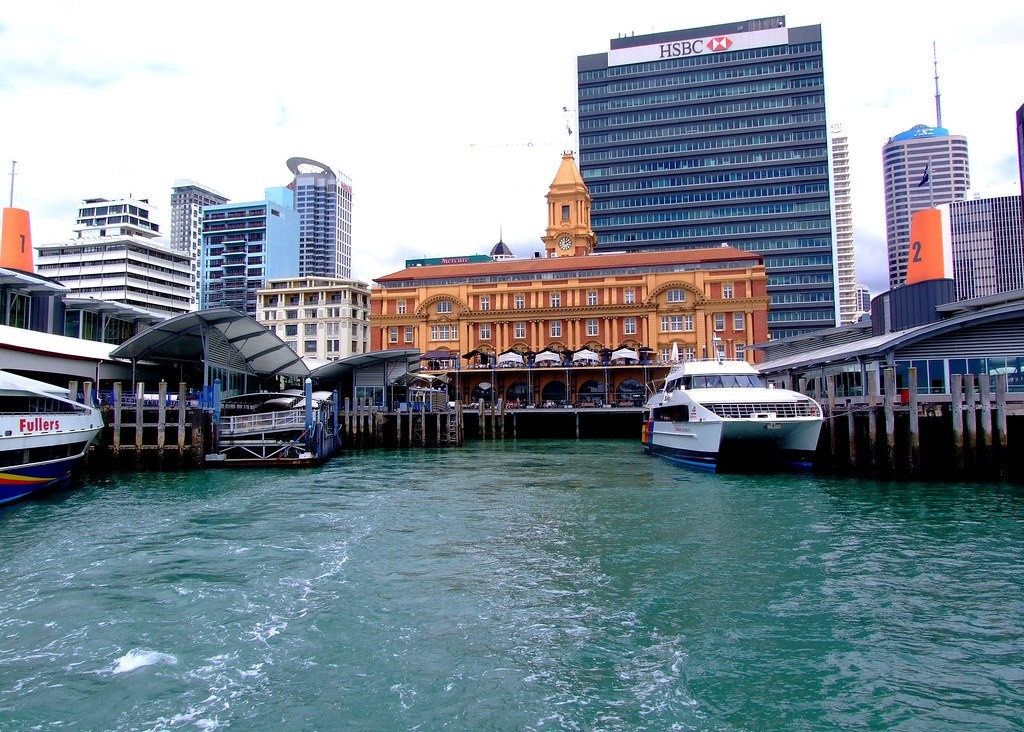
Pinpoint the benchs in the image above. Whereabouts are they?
[581,403,595,407]
[620,402,635,407]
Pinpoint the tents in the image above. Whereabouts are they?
[534,347,560,362]
[572,346,599,361]
[611,345,639,361]
[498,348,524,363]
[421,350,451,360]
[462,349,491,360]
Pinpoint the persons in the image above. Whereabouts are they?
[536,401,554,408]
[594,398,603,408]
[506,397,526,409]
[475,361,489,368]
[499,361,513,368]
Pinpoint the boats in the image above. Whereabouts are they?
[0,366,106,509]
[639,330,824,473]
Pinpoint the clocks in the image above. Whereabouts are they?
[558,237,572,251]
